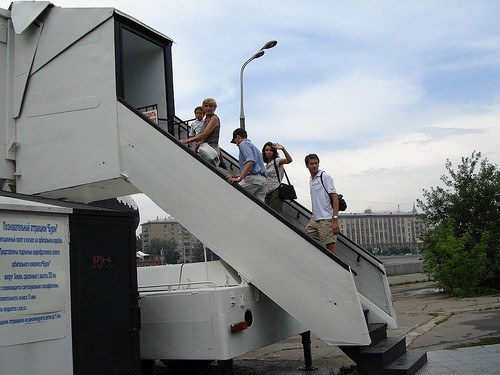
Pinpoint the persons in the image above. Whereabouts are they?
[302,154,339,255]
[226,128,267,203]
[179,97,220,168]
[262,141,292,212]
[189,106,206,149]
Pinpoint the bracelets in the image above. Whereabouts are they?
[189,138,191,142]
[237,177,242,181]
[332,216,338,218]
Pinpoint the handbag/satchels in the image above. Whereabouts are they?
[330,194,347,211]
[197,143,218,163]
[278,183,297,201]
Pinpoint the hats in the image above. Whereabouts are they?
[230,128,246,143]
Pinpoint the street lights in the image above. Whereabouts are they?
[239,40,278,129]
